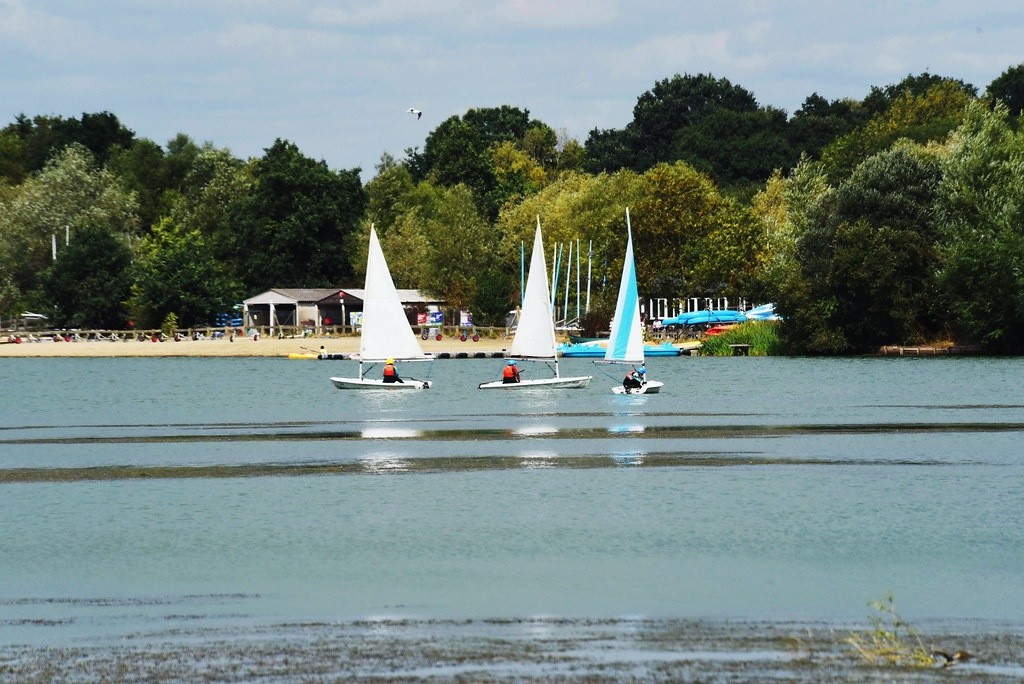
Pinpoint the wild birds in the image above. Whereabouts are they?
[406,107,422,120]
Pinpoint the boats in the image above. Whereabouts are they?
[560,332,682,357]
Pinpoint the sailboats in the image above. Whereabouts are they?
[329,222,434,390]
[479,213,593,391]
[594,207,663,396]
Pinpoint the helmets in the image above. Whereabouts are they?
[508,359,516,366]
[386,357,393,364]
[638,367,646,374]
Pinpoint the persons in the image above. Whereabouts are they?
[383,359,404,383]
[503,360,520,383]
[320,345,328,355]
